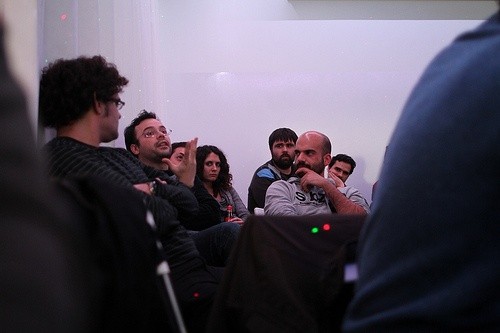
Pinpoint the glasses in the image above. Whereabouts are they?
[137,128,172,142]
[105,97,126,110]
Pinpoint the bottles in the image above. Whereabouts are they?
[225,205,234,224]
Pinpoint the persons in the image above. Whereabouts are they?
[196,146,248,231]
[343,0,500,333]
[247,128,298,216]
[264,131,370,217]
[327,154,356,188]
[38,56,244,333]
[0,18,84,333]
[168,142,219,231]
[123,109,242,293]
